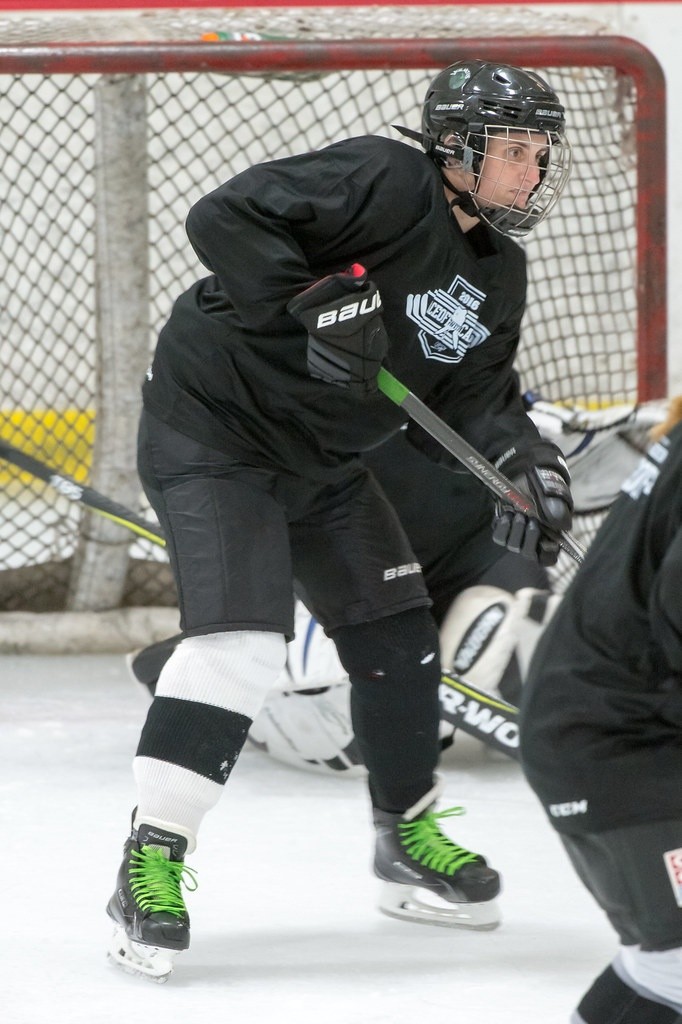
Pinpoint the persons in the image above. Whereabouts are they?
[520,420,682,1024]
[126,389,669,780]
[106,59,577,983]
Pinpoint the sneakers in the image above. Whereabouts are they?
[100,805,198,984]
[370,777,502,931]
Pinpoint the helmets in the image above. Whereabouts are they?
[419,57,573,238]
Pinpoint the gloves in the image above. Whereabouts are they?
[483,437,573,566]
[288,264,391,400]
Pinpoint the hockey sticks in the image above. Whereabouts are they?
[0,440,526,763]
[377,364,587,564]
